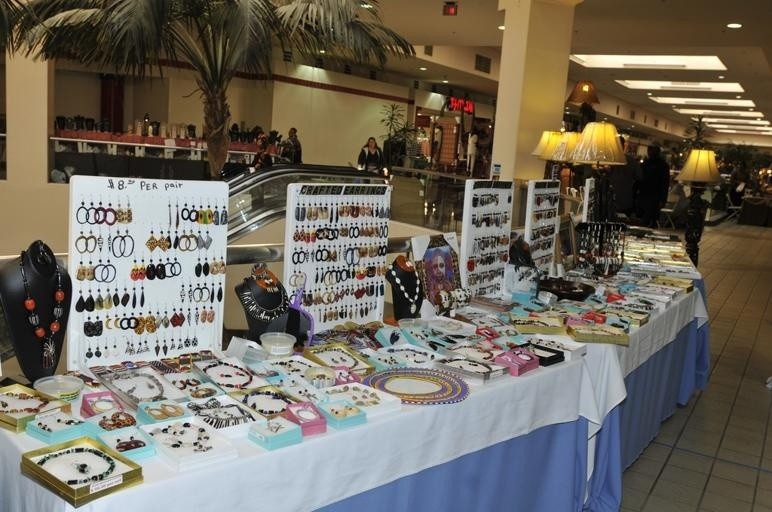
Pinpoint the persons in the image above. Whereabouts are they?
[281,128,302,163]
[425,248,455,306]
[464,125,480,178]
[244,137,273,212]
[356,136,383,174]
[730,160,744,177]
[427,122,442,163]
[596,133,683,229]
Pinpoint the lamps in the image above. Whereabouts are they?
[532,121,627,255]
[567,80,600,103]
[675,150,722,268]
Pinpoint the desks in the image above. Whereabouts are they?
[0,240,711,512]
[55,128,277,164]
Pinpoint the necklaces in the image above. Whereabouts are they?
[17,242,65,369]
[240,276,290,322]
[509,276,691,340]
[575,221,626,278]
[0,310,581,485]
[388,260,420,315]
